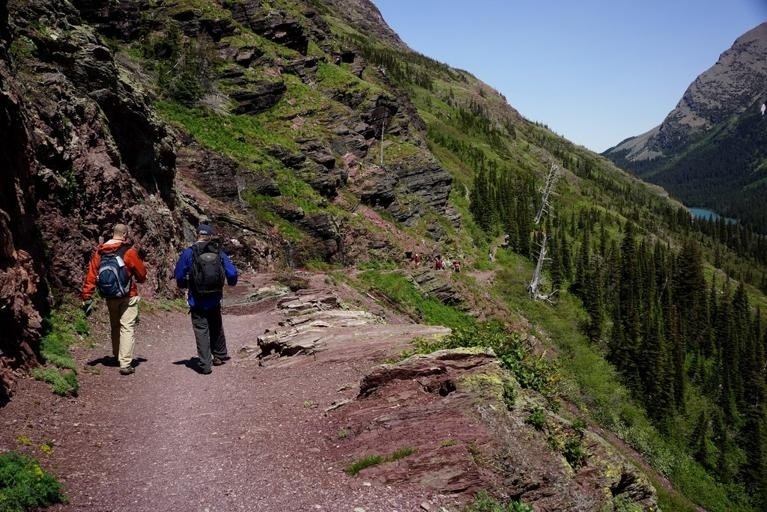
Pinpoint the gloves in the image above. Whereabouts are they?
[84,300,96,317]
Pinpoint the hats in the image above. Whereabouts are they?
[196,224,212,235]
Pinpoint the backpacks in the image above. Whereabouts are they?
[96,254,131,298]
[193,253,225,298]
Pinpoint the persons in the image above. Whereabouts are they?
[82,224,147,376]
[174,225,238,375]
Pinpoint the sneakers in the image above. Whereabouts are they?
[120,367,134,375]
[191,358,211,374]
[214,358,222,365]
